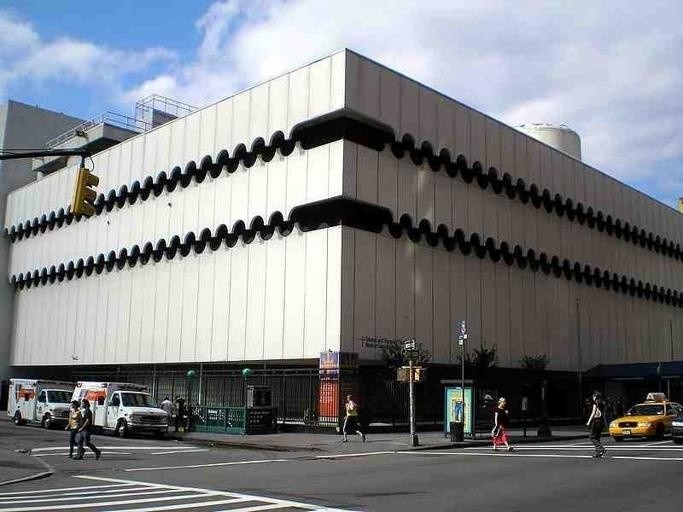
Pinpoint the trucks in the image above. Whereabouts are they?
[69,379,170,439]
[6,377,73,430]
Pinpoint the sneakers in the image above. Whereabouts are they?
[73,448,100,459]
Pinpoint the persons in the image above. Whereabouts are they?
[581,394,625,427]
[491,396,513,452]
[585,390,606,459]
[341,394,365,443]
[73,399,101,461]
[173,395,188,433]
[64,400,82,458]
[161,396,173,434]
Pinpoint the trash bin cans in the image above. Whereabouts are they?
[450,422,463,442]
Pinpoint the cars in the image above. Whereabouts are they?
[608,392,683,442]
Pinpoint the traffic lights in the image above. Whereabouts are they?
[69,167,100,217]
